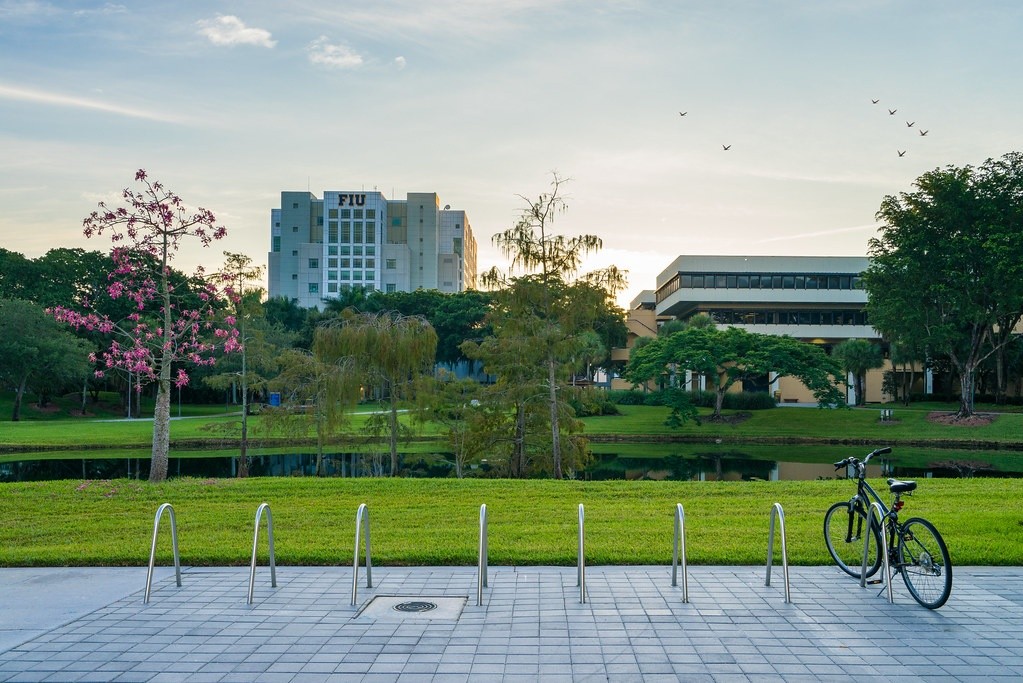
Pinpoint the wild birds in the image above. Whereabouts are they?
[872,99,879,104]
[679,112,687,116]
[920,130,928,136]
[906,121,915,127]
[722,144,731,150]
[889,109,897,115]
[898,151,906,157]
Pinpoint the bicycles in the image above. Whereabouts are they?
[823,446,953,609]
